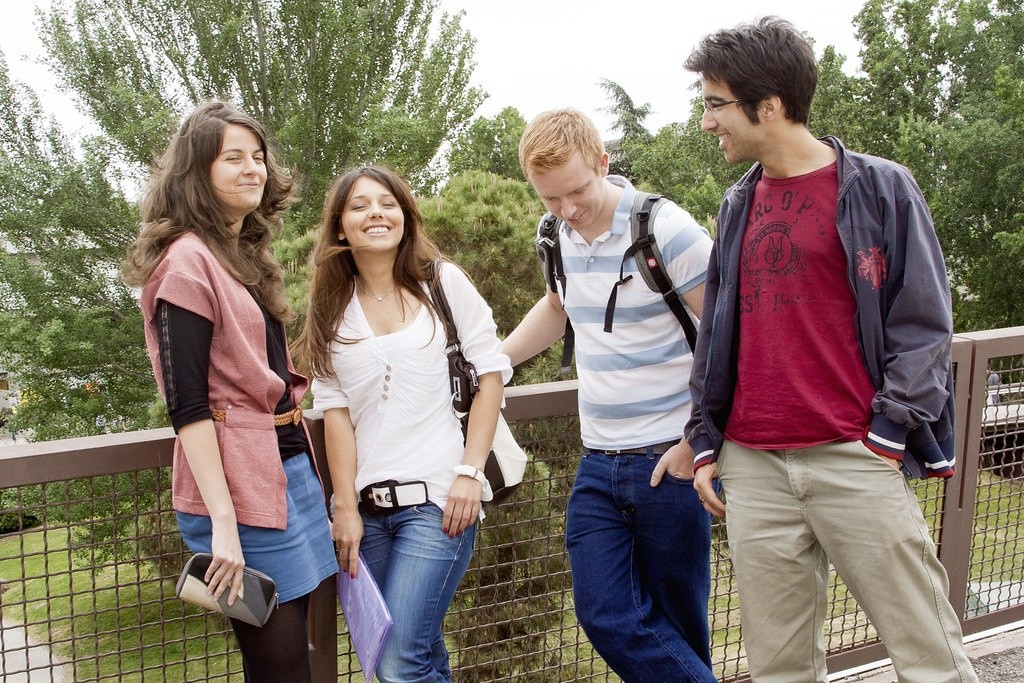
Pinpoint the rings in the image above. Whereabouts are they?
[699,497,705,505]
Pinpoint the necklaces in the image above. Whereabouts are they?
[353,273,398,303]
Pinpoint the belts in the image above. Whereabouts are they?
[360,482,430,509]
[208,402,305,426]
[594,439,682,455]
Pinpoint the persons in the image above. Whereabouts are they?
[679,16,989,683]
[117,98,342,683]
[498,106,728,683]
[288,162,515,683]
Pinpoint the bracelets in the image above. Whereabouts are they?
[452,463,487,489]
